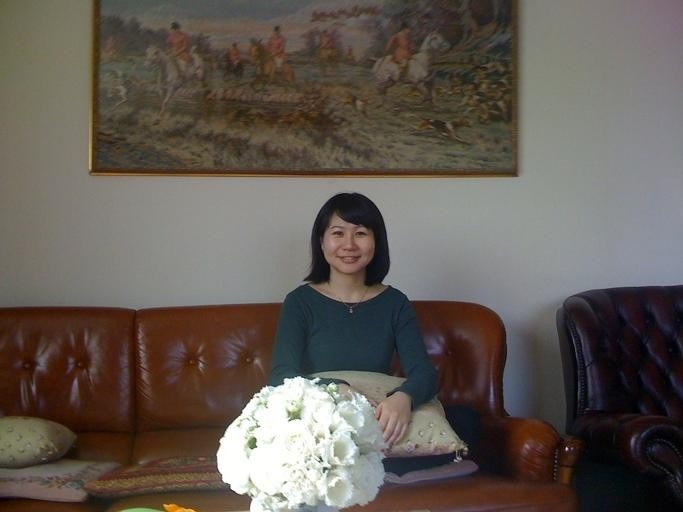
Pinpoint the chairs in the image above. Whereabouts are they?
[557,284,683,512]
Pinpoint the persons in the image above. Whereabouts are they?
[267,191,457,477]
[162,22,418,82]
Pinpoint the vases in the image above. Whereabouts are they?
[249,499,341,512]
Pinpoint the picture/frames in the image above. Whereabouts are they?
[88,0,520,180]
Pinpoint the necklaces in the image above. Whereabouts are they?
[328,282,368,313]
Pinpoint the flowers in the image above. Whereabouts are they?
[216,376,386,510]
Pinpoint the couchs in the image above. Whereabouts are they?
[0,299,584,512]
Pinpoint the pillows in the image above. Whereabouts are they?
[310,371,469,459]
[0,416,78,468]
